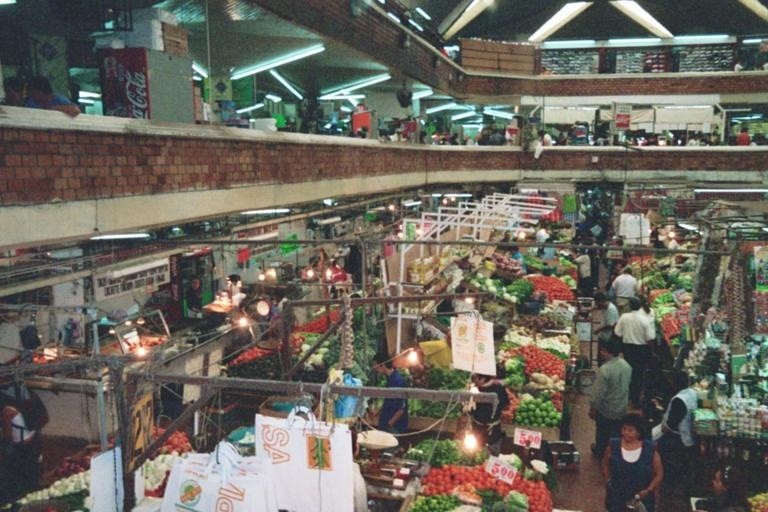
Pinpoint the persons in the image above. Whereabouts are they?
[540,67,552,75]
[578,291,623,369]
[652,369,698,493]
[510,246,526,273]
[469,367,510,453]
[599,414,665,512]
[499,231,518,252]
[450,122,768,148]
[587,343,633,461]
[0,77,27,107]
[572,181,662,349]
[695,464,753,512]
[542,238,557,260]
[451,285,486,319]
[536,223,549,253]
[186,278,203,318]
[1,390,48,507]
[21,76,82,116]
[372,354,410,445]
[327,258,348,298]
[734,60,746,71]
[614,296,658,408]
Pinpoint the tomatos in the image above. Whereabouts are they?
[407,463,554,512]
[55,425,194,479]
[509,344,565,379]
[528,275,575,302]
[228,333,305,365]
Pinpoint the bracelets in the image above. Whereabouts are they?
[642,488,651,496]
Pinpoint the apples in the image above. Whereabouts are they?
[513,399,563,428]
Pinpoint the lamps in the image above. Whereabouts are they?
[191,40,392,116]
